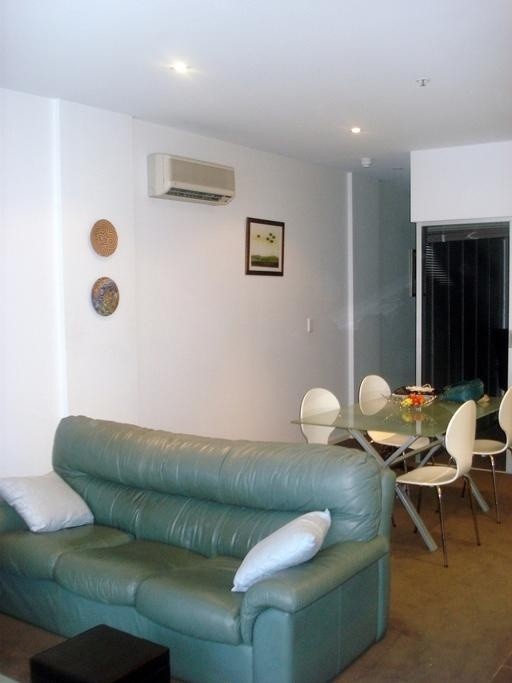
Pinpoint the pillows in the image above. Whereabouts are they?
[2,470,95,531]
[230,508,331,591]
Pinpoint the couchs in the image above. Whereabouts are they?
[1,415,396,683]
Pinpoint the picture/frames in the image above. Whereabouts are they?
[244,217,285,276]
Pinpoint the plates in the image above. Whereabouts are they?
[383,394,436,405]
[379,409,432,425]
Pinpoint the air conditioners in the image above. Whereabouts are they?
[145,153,235,205]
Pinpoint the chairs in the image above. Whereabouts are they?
[359,374,435,498]
[395,400,481,567]
[435,386,512,524]
[299,388,396,527]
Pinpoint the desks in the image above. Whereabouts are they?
[291,394,503,552]
